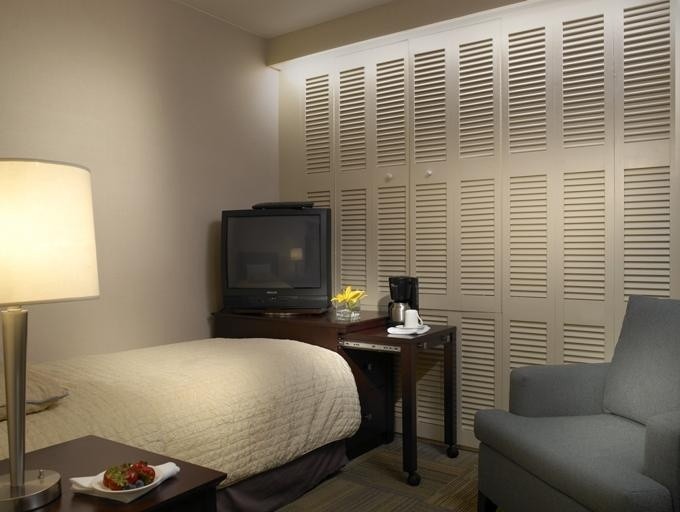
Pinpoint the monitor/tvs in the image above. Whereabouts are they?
[221,209,331,309]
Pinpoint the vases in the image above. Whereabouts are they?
[335,306,363,324]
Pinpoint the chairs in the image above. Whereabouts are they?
[471,294,680,512]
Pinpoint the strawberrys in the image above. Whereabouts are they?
[103,461,155,490]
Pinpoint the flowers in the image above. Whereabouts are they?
[331,285,365,307]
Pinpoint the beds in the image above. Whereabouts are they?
[0,337,363,512]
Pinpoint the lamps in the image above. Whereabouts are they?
[0,157,100,511]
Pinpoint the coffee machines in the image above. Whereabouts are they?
[388,274,420,324]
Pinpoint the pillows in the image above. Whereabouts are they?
[0,361,70,424]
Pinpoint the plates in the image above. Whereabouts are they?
[91,464,164,494]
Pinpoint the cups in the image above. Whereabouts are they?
[405,309,424,328]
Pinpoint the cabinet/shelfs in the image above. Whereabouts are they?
[210,308,392,460]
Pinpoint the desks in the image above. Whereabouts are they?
[340,322,459,488]
[0,434,229,510]
